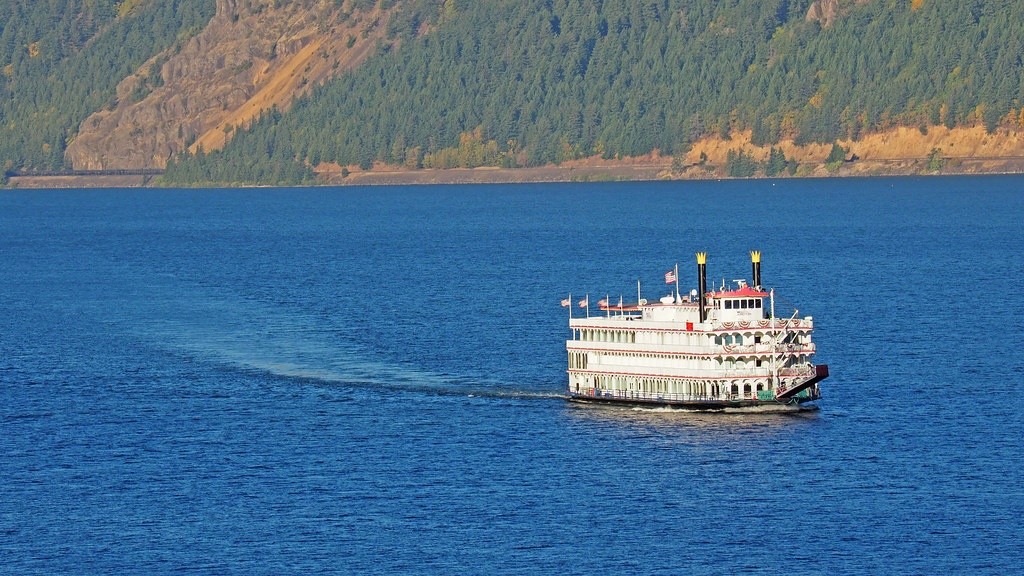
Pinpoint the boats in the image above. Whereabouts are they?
[565,251,830,412]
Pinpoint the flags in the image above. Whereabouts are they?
[578,300,586,308]
[560,298,569,307]
[617,298,622,309]
[597,299,608,307]
[665,269,679,284]
